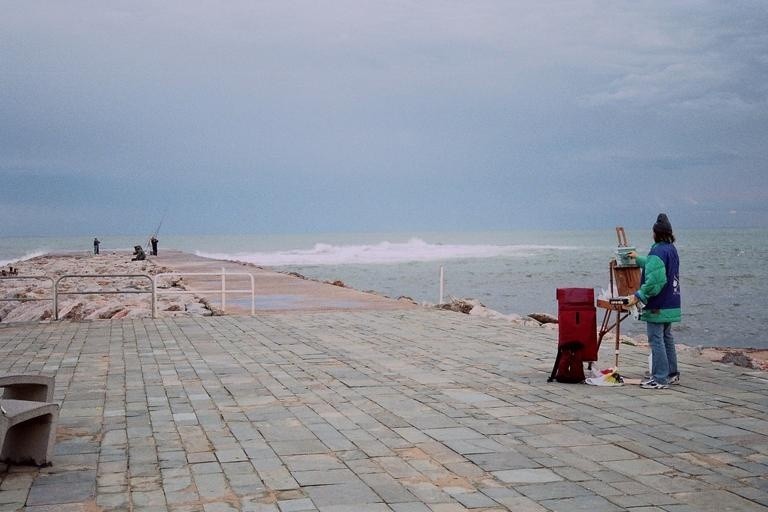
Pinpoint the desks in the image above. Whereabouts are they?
[588,298,631,370]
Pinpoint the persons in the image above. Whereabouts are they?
[150,237,159,256]
[94,238,100,254]
[626,212,681,389]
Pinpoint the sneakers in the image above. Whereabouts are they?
[640,375,680,389]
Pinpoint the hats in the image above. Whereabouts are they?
[654,213,672,232]
